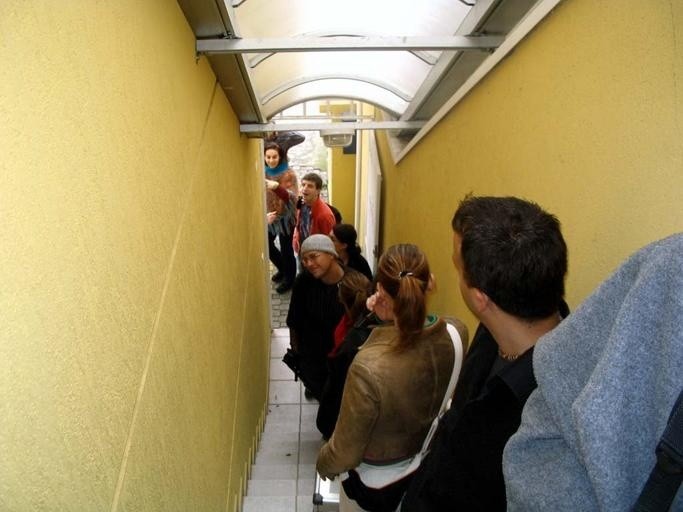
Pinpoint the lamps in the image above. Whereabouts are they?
[320,128,355,148]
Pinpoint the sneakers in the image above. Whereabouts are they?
[276,282,290,293]
[272,271,283,281]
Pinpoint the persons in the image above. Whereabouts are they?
[399,188,570,511]
[264,130,378,441]
[316,243,469,511]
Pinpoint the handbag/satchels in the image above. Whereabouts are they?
[356,451,428,489]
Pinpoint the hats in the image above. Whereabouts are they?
[301,235,338,257]
